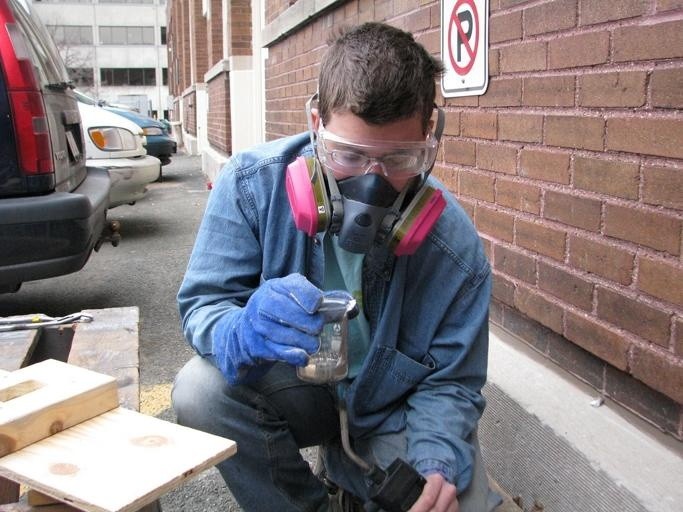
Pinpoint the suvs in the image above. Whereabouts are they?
[97,99,178,166]
[0,0,120,296]
[72,91,162,210]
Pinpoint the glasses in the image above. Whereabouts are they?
[318,116,440,177]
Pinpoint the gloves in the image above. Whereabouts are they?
[210,273,360,389]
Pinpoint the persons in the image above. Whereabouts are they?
[170,22,507,511]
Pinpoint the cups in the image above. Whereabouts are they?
[296,290,357,385]
